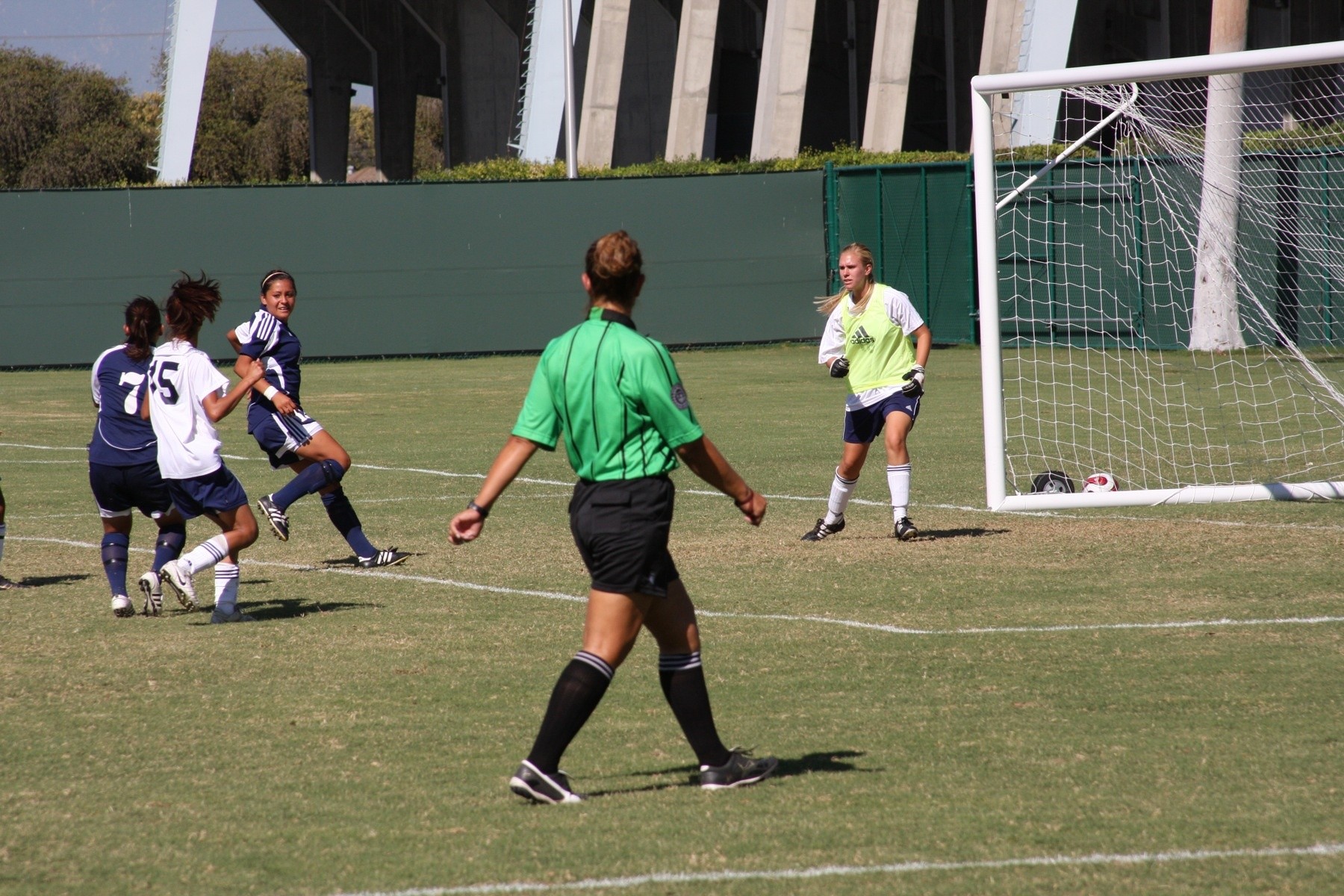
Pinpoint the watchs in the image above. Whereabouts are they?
[466,500,488,520]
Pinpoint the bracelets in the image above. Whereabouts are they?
[734,489,754,506]
[264,385,279,401]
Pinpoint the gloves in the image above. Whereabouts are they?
[829,355,850,378]
[901,364,926,397]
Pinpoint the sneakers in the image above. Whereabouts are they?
[698,742,780,790]
[801,515,845,541]
[358,546,410,568]
[211,607,259,623]
[0,574,35,590]
[894,517,918,540]
[257,494,289,542]
[158,561,201,612]
[507,759,588,808]
[112,594,136,617]
[138,571,162,616]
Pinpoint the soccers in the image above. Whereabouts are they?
[1081,472,1119,493]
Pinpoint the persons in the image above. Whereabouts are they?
[148,267,265,623]
[800,244,932,541]
[89,295,187,618]
[226,269,411,570]
[450,231,780,808]
[0,485,13,590]
